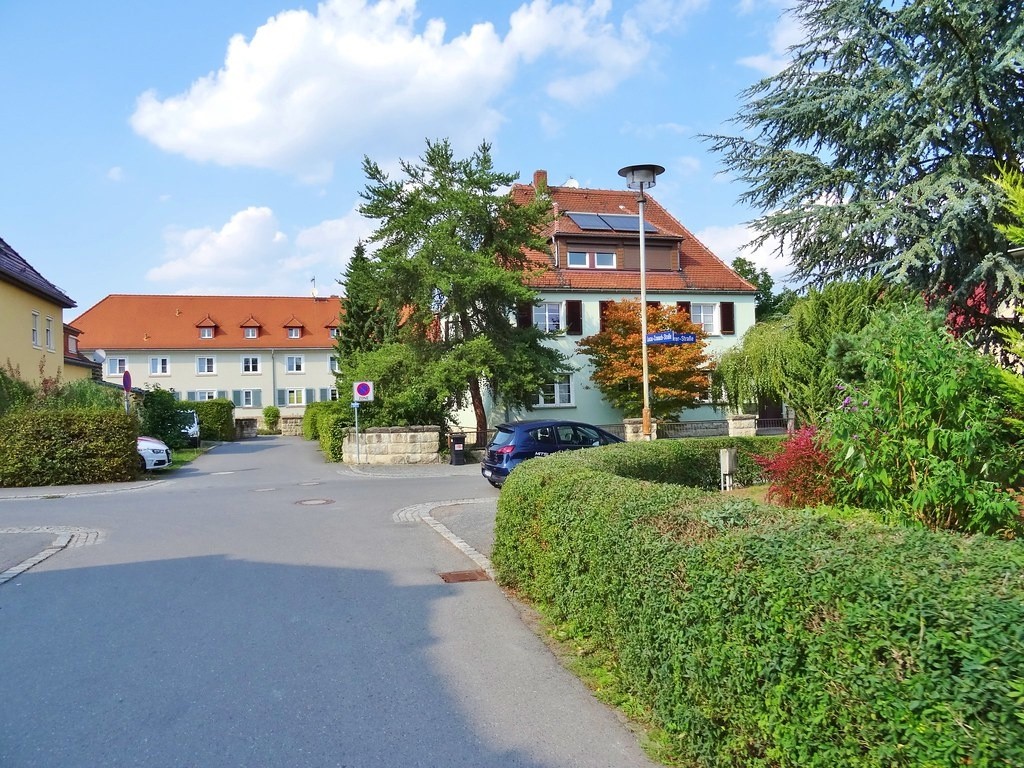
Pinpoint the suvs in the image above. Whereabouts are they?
[176,408,203,448]
[481,419,628,491]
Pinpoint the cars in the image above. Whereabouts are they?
[135,434,174,475]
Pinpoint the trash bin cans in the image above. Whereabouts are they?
[448,433,466,465]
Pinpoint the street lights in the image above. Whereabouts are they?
[618,164,679,439]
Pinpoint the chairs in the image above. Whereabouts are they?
[539,429,555,440]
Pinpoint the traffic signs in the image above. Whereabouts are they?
[673,333,697,344]
[645,329,675,345]
